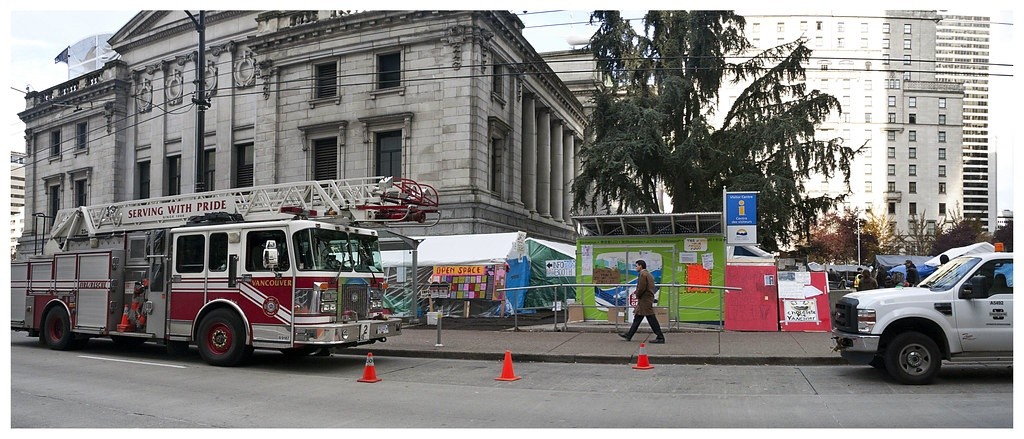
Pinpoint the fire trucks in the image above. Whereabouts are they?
[12,174,444,372]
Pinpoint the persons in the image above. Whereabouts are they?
[618,260,665,344]
[934,254,950,272]
[903,260,920,287]
[829,268,840,281]
[853,265,899,291]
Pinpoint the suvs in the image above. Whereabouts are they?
[829,253,1013,387]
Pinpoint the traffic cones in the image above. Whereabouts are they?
[492,349,522,383]
[356,351,384,385]
[633,340,655,370]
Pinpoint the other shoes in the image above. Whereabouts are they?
[649,338,665,344]
[617,333,631,341]
